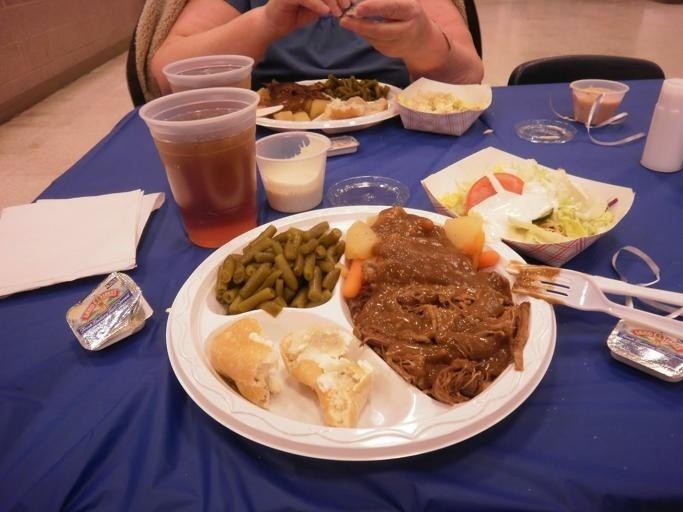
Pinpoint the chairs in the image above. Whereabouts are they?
[508,55,665,86]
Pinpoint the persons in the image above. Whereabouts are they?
[120,0,486,99]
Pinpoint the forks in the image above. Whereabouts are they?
[512,267,683,341]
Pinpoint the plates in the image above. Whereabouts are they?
[254,79,404,131]
[166,205,557,462]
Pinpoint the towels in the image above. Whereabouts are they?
[0,184,167,300]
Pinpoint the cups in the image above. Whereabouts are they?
[255,131,332,213]
[161,55,254,94]
[139,86,260,248]
[571,78,629,126]
